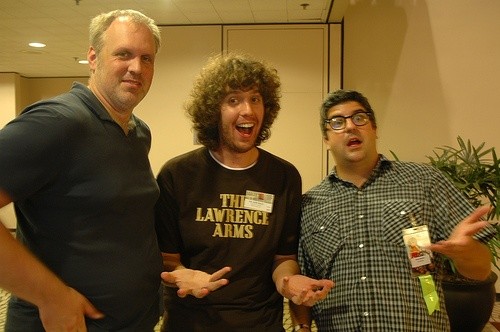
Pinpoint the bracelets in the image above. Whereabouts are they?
[293,324,311,332]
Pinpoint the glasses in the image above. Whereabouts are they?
[325,111,371,131]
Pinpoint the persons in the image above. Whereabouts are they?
[408,238,423,258]
[289,89,498,332]
[156,49,335,332]
[0,10,165,332]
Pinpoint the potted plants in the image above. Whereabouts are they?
[389,136,500,332]
